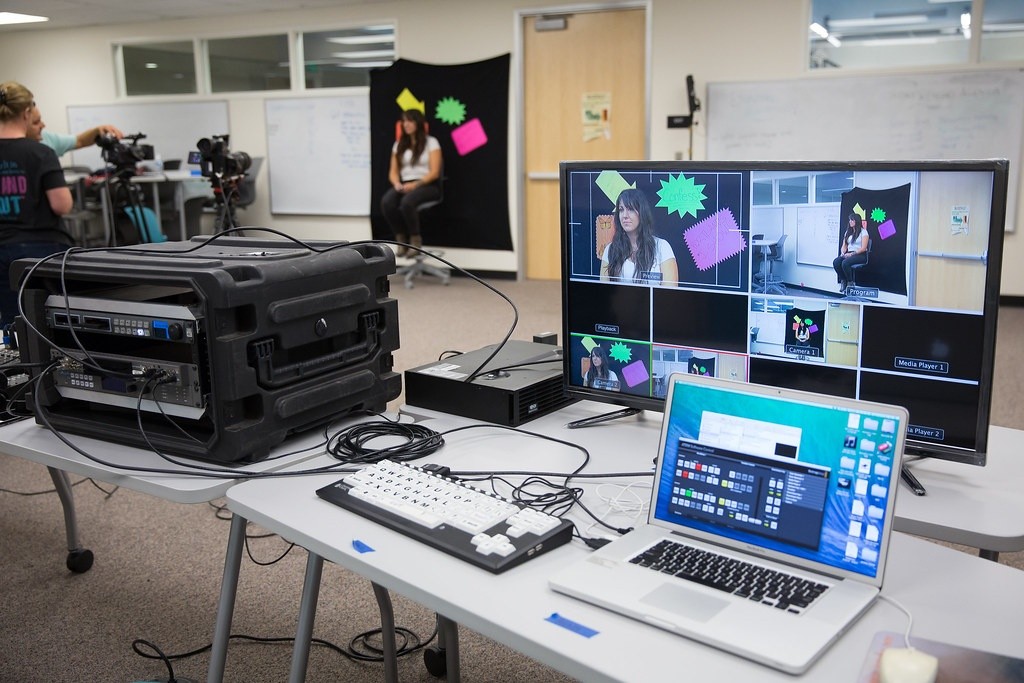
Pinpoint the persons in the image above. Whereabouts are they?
[600,188,678,289]
[833,213,869,293]
[380,109,442,259]
[583,347,620,392]
[26,101,126,157]
[0,82,73,330]
[795,320,811,347]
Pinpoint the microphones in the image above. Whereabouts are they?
[122,134,147,139]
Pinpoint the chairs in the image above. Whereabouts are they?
[796,338,811,360]
[652,373,667,394]
[387,157,450,290]
[840,239,872,302]
[752,234,788,295]
[751,327,760,355]
[203,157,263,237]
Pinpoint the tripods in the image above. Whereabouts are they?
[212,181,244,238]
[110,168,151,246]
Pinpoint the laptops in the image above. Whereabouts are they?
[549,373,909,676]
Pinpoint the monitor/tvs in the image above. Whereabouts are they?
[559,160,1010,495]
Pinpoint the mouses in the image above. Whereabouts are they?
[879,648,938,683]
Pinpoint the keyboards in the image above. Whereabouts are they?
[315,458,573,575]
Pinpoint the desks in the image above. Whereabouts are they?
[654,375,662,397]
[399,381,1024,677]
[63,171,166,247]
[0,412,413,683]
[752,240,778,294]
[164,169,215,242]
[224,418,1023,683]
